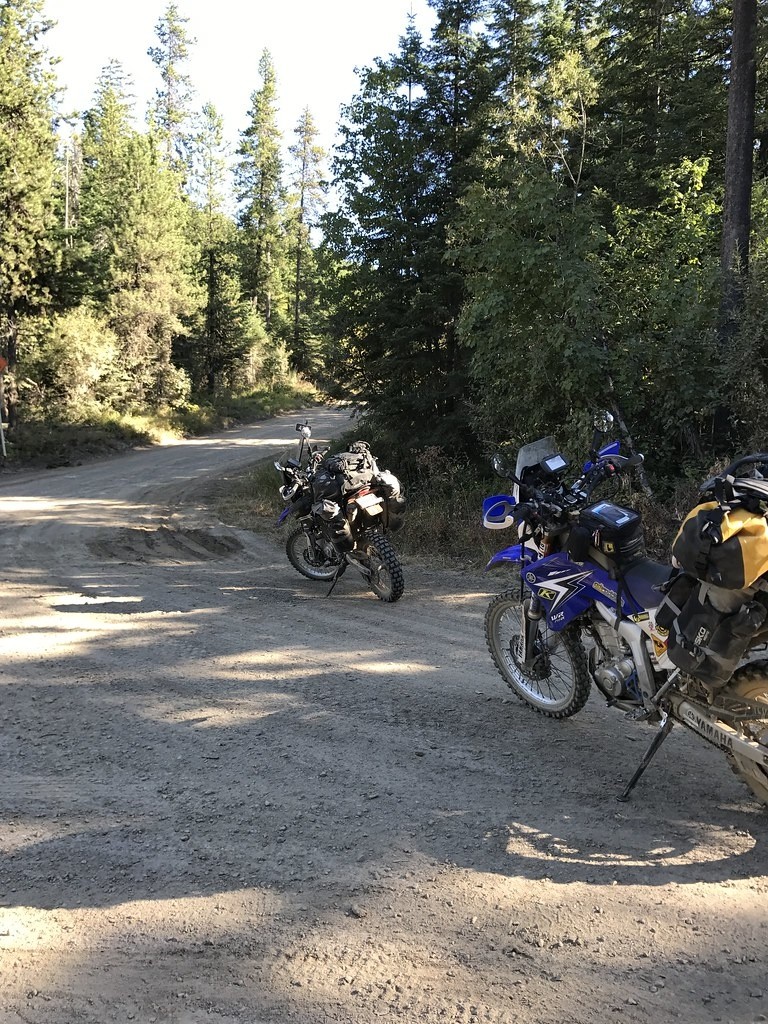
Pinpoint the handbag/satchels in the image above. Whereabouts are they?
[657,453,767,684]
[566,502,643,562]
[312,442,408,550]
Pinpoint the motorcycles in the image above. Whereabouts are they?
[272,419,406,603]
[481,408,768,811]
